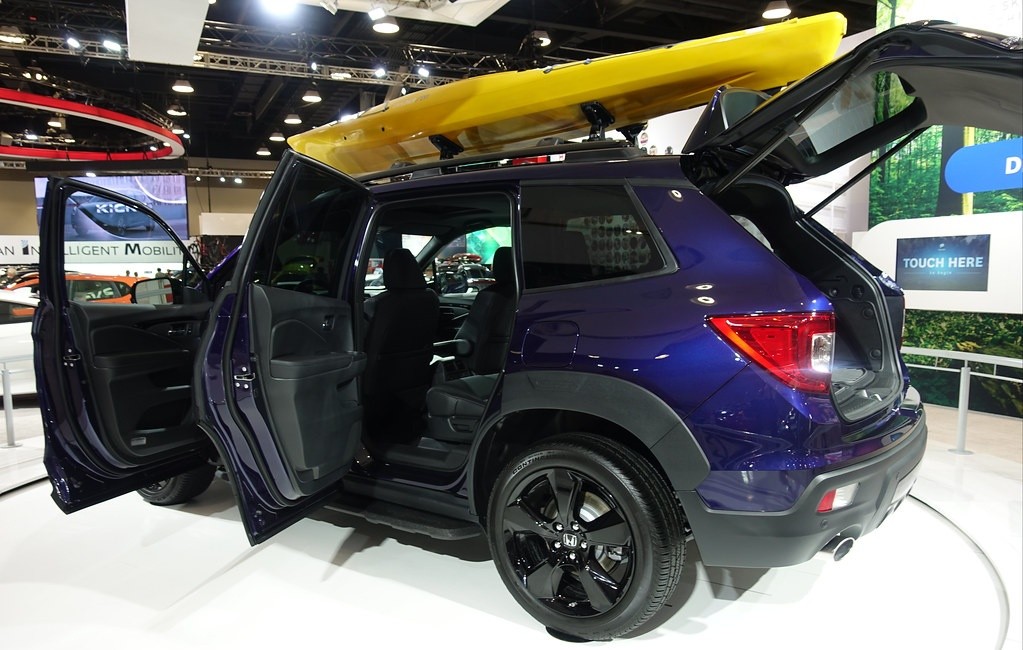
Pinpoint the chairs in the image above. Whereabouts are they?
[361,246,440,409]
[453,245,516,373]
[425,232,594,444]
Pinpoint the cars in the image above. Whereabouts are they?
[365,253,496,294]
[0,264,152,323]
[71,189,155,237]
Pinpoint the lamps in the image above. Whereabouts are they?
[256,147,271,155]
[761,0,791,19]
[269,133,285,141]
[302,90,322,102]
[373,16,400,34]
[47,117,61,127]
[284,114,302,124]
[166,105,187,116]
[532,31,551,47]
[173,79,194,93]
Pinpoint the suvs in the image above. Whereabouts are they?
[32,11,1023,641]
